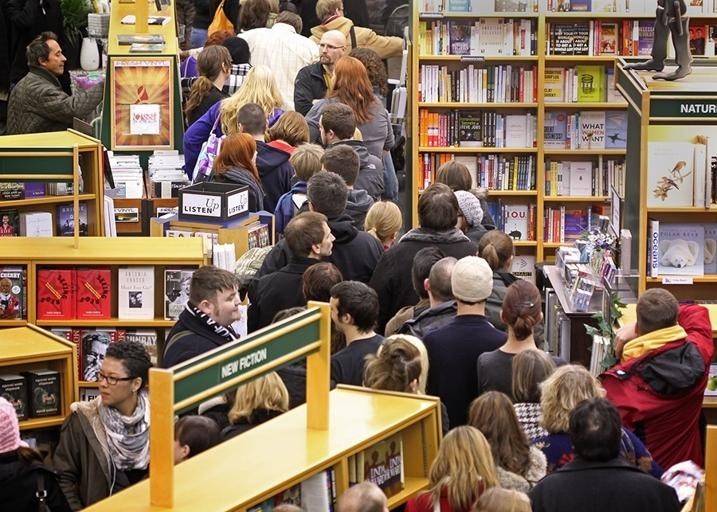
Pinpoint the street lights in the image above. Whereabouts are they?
[192,134,221,184]
[208,6,234,38]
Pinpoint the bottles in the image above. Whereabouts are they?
[0,397,22,452]
[453,189,485,226]
[450,254,493,303]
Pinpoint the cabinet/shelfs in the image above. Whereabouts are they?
[404,0,717,512]
[79,379,447,512]
[0,1,277,450]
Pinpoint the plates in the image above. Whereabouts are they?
[79,379,447,512]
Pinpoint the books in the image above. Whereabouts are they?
[391,86,407,123]
[249,431,405,509]
[546,0,714,378]
[399,49,408,86]
[72,271,77,319]
[417,3,541,193]
[3,152,194,458]
[512,254,536,285]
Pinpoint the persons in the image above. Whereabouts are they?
[689,29,694,47]
[0,266,286,511]
[691,28,705,55]
[329,160,715,510]
[184,1,412,338]
[1,1,105,135]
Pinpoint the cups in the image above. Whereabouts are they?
[94,370,136,387]
[319,41,343,51]
